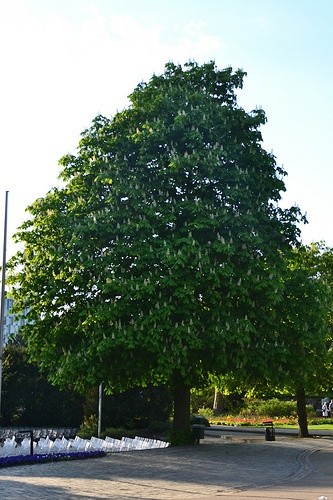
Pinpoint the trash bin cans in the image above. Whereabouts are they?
[191,416,205,444]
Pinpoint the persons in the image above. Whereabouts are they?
[321,401,330,418]
[328,399,333,418]
